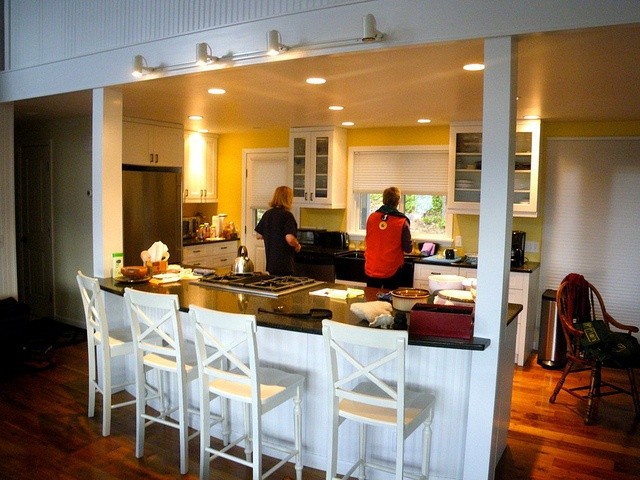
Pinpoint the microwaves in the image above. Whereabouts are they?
[294,229,346,254]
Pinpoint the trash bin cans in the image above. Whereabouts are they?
[537,288,569,369]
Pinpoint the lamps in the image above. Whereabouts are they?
[196,42,220,67]
[362,13,386,43]
[131,55,156,76]
[265,29,290,57]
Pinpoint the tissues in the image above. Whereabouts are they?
[146,240,170,276]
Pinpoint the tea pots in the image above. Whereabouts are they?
[233,245,254,277]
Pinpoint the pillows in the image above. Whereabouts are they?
[574,321,613,346]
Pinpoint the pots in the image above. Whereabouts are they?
[388,287,431,312]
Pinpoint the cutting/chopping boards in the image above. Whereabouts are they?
[415,251,464,264]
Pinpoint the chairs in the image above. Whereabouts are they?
[549,272,640,427]
[322,318,436,480]
[122,283,231,475]
[187,304,304,480]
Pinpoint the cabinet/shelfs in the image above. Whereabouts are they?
[415,263,460,296]
[463,270,540,367]
[183,135,219,203]
[180,238,241,269]
[287,127,346,210]
[123,119,185,167]
[446,121,538,217]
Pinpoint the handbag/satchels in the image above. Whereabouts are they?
[602,332,639,369]
[572,320,610,358]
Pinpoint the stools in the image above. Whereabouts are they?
[77,270,165,437]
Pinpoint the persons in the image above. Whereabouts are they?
[364,187,413,290]
[254,186,302,276]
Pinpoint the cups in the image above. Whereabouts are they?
[445,249,455,260]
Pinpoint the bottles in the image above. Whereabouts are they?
[204,222,210,238]
[199,225,205,241]
[210,226,216,239]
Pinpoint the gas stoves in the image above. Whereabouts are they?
[190,270,327,300]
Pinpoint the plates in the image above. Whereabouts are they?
[454,178,474,189]
[114,276,152,284]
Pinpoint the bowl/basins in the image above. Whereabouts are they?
[121,265,147,279]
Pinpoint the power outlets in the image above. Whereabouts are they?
[455,236,462,247]
[533,243,539,252]
[525,242,532,252]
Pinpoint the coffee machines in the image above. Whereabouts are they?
[507,231,527,267]
